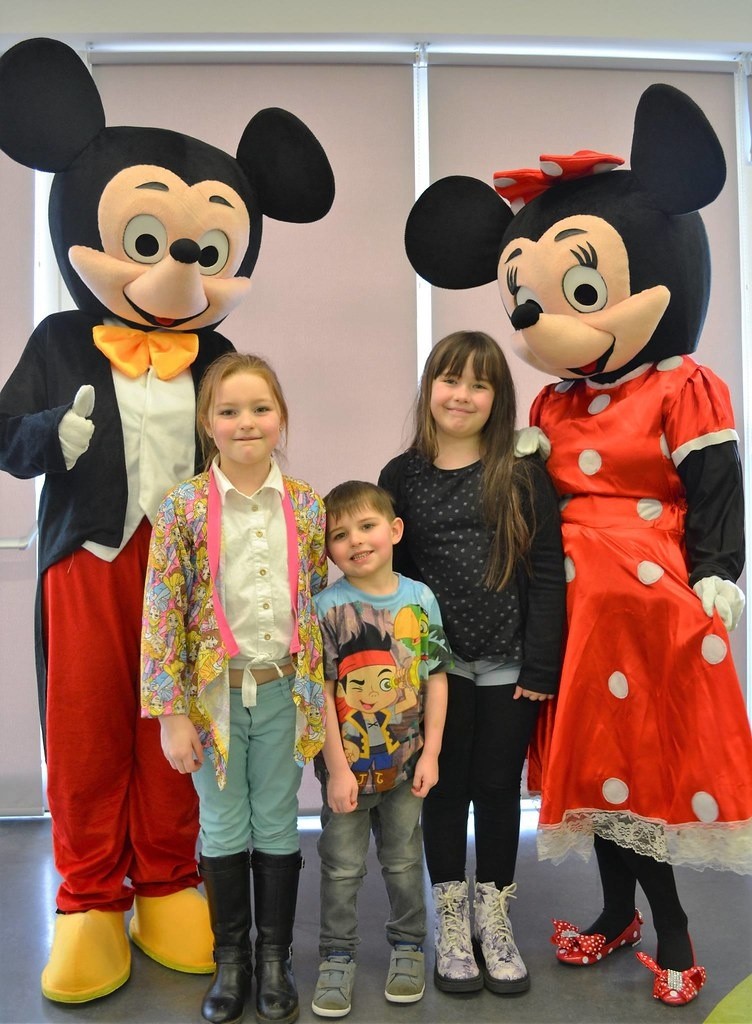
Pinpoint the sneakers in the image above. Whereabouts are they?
[384,944,426,1004]
[311,955,358,1018]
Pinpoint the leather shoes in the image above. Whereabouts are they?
[635,930,704,1008]
[552,909,643,966]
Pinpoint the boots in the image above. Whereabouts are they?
[195,850,251,1024]
[432,875,484,994]
[473,882,531,994]
[253,850,305,1024]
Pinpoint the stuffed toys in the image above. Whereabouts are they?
[0,37,334,1005]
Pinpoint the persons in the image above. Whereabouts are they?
[140,355,330,1024]
[380,328,565,993]
[405,82,751,1008]
[307,481,452,1016]
